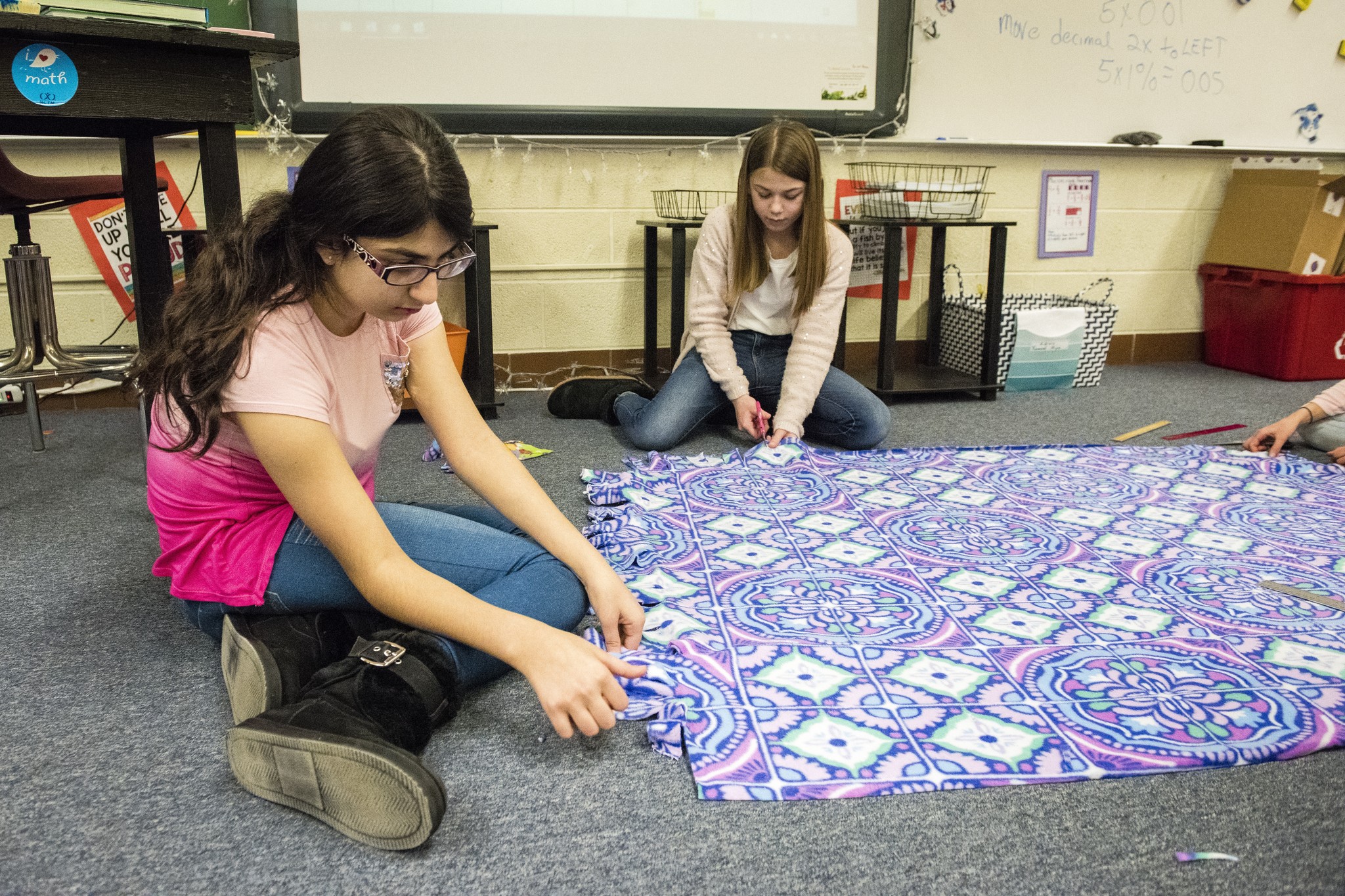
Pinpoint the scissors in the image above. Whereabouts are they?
[755,401,765,446]
[1206,439,1295,450]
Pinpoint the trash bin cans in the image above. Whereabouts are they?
[1198,264,1345,380]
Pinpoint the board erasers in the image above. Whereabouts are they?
[1192,140,1223,147]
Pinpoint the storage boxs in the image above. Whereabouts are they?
[1198,264,1345,380]
[940,264,1119,388]
[1204,156,1345,274]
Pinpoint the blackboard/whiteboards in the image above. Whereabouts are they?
[897,0,1345,158]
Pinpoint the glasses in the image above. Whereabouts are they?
[342,233,476,286]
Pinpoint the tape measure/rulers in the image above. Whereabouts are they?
[1161,424,1247,440]
[1110,420,1170,442]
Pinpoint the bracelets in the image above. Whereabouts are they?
[1300,407,1313,424]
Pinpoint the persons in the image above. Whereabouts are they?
[130,105,646,848]
[1243,379,1345,466]
[547,118,891,451]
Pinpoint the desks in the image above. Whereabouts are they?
[0,13,299,457]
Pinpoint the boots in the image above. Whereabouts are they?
[702,400,737,426]
[224,611,411,726]
[548,376,653,426]
[230,635,461,850]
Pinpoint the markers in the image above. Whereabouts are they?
[937,137,971,141]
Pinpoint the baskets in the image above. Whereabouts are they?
[939,263,1118,387]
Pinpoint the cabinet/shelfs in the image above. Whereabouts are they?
[636,220,704,386]
[828,219,1017,404]
[162,227,504,417]
